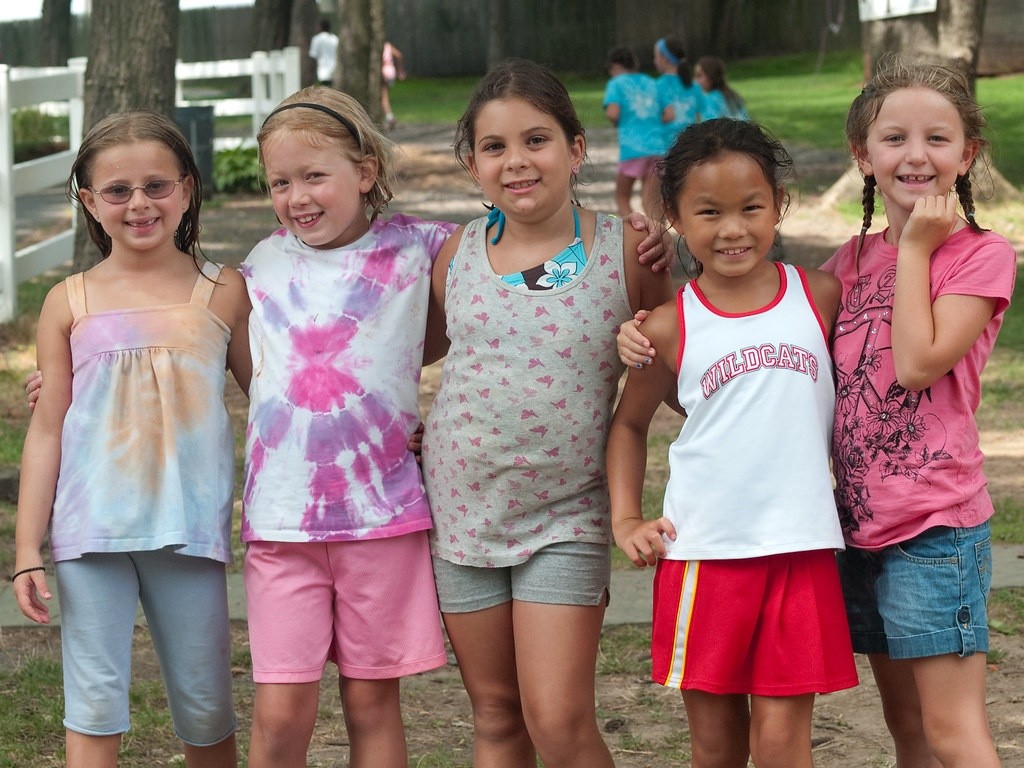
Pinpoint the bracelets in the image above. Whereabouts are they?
[12,567,45,584]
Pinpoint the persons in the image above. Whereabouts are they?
[309,17,339,87]
[380,42,406,130]
[421,58,690,768]
[607,117,859,768]
[24,85,678,768]
[11,109,428,768]
[617,61,1019,768]
[602,36,750,227]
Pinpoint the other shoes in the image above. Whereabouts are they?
[383,118,397,133]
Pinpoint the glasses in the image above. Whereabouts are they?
[87,174,187,205]
